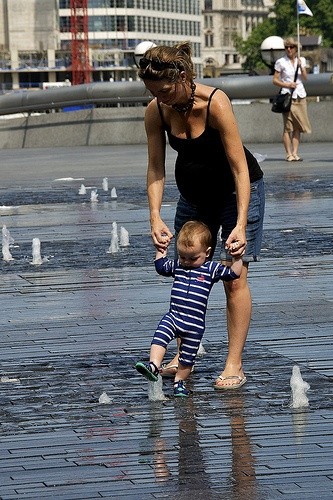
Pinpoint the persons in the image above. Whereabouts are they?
[138,43,265,390]
[135,221,243,396]
[272,37,311,162]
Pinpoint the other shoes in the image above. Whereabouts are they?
[136,361,159,382]
[173,380,190,398]
[285,154,303,161]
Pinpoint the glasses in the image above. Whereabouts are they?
[285,46,296,49]
[139,59,182,73]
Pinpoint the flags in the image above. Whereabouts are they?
[297,0,313,16]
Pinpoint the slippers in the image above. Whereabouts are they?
[160,363,194,377]
[214,375,248,390]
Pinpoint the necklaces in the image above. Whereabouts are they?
[170,78,196,117]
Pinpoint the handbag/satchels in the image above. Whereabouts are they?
[271,93,292,114]
